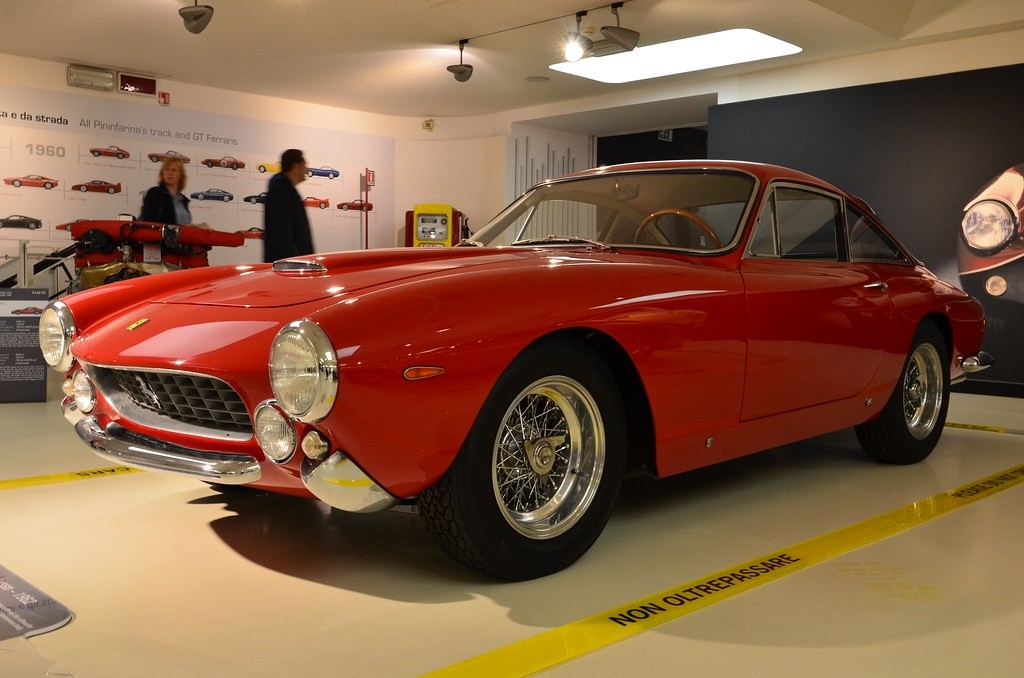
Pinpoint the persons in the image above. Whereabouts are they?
[264,149,315,263]
[139,156,192,225]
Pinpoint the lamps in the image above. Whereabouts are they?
[447,39,473,82]
[601,3,640,52]
[178,0,213,34]
[562,12,594,63]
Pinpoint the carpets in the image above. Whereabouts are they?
[0,565,73,642]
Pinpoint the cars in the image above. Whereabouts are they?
[953,165,1024,325]
[255,162,281,174]
[191,188,234,202]
[4,174,58,189]
[201,155,245,171]
[0,214,42,231]
[90,145,130,160]
[236,227,265,240]
[303,196,330,209]
[71,179,121,195]
[243,192,267,204]
[305,165,340,180]
[148,150,191,164]
[337,199,373,212]
[37,158,995,584]
